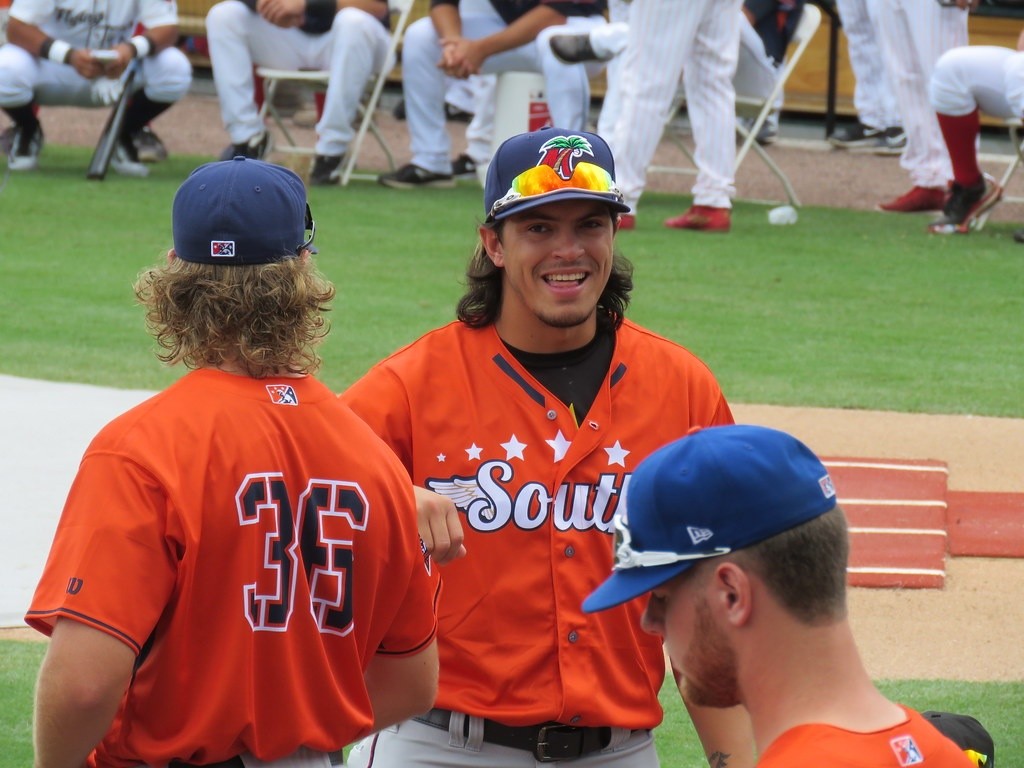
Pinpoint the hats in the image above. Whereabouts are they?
[484,126,630,221]
[583,423,836,613]
[171,155,307,264]
[920,710,993,768]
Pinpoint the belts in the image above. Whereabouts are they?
[204,748,344,768]
[412,707,639,762]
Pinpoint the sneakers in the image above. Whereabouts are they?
[220,132,270,159]
[927,171,1002,234]
[109,138,148,177]
[663,205,732,232]
[828,123,886,149]
[379,162,456,191]
[877,186,945,211]
[453,155,475,177]
[885,126,907,147]
[616,217,637,230]
[735,112,779,138]
[134,129,169,162]
[548,32,595,64]
[309,153,344,184]
[6,121,43,170]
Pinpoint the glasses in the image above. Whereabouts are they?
[295,203,315,252]
[487,162,624,217]
[609,515,732,573]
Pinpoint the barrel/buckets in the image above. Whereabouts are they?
[490,69,554,161]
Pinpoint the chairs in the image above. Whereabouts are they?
[964,117,1024,235]
[252,2,416,190]
[642,4,823,216]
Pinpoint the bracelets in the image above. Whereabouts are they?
[39,38,72,62]
[125,32,156,60]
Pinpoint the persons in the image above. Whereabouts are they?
[0,0,1024,235]
[23,157,440,768]
[336,125,759,768]
[582,424,985,768]
[922,711,995,768]
